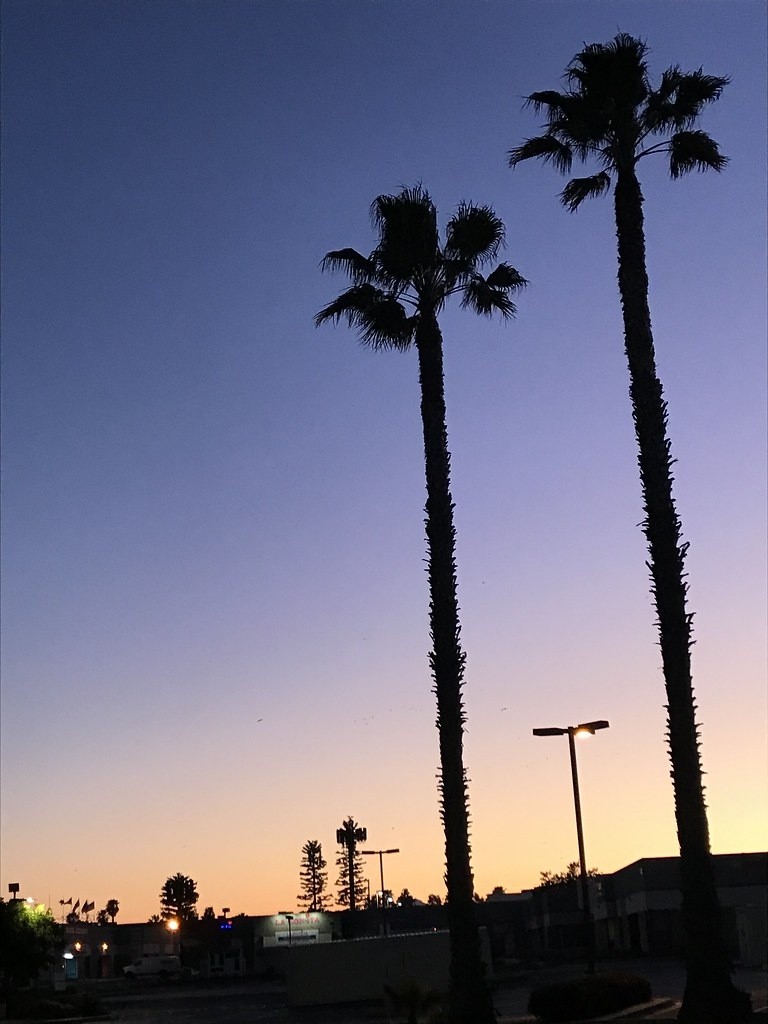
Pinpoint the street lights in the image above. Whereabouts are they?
[362,848,400,935]
[286,916,294,946]
[530,718,610,972]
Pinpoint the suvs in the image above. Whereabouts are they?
[123,956,182,980]
